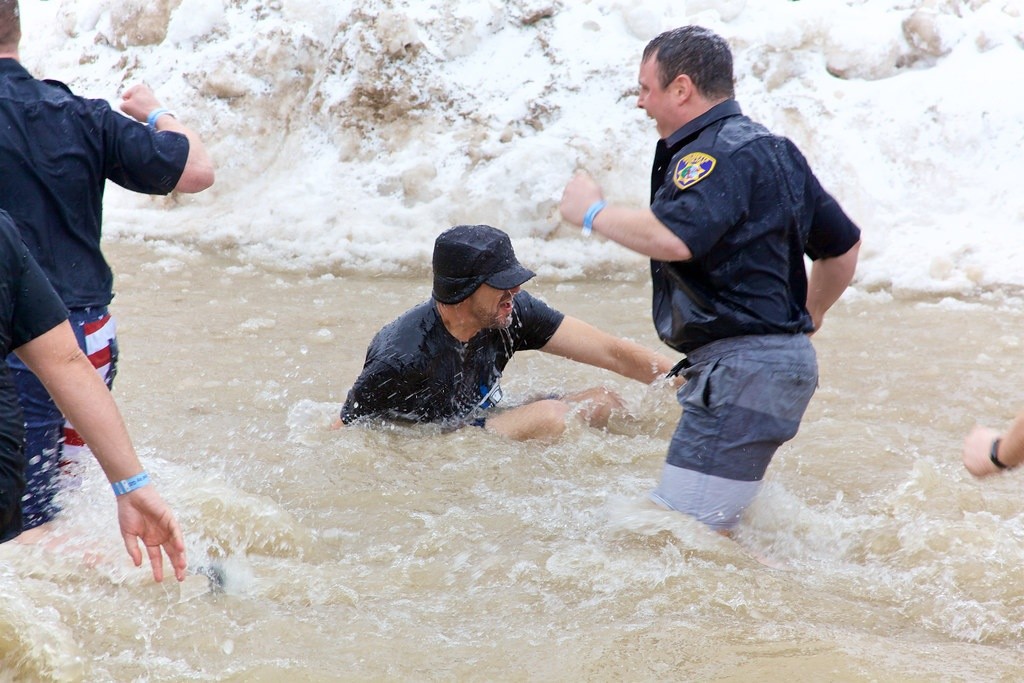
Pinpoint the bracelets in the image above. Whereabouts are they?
[146,106,180,133]
[990,437,1017,472]
[580,200,607,237]
[111,471,153,497]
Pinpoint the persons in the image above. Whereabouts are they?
[0,2,216,534]
[560,24,862,544]
[960,408,1024,479]
[330,223,688,450]
[0,199,188,584]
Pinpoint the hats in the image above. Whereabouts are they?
[429,225,535,305]
